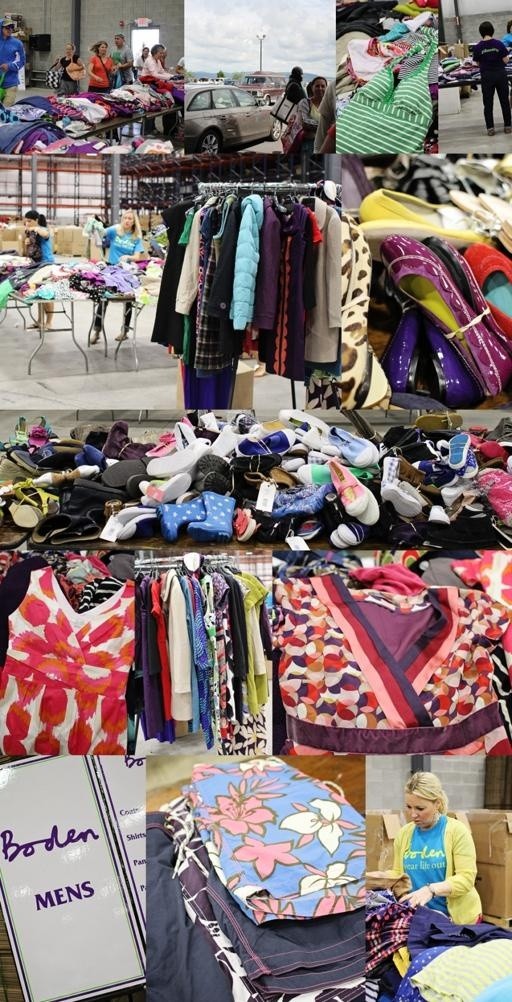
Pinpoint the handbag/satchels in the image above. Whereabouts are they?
[66,53,86,80]
[269,82,302,125]
[45,58,65,89]
[281,97,311,156]
[106,69,118,88]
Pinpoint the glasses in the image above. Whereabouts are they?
[4,24,14,29]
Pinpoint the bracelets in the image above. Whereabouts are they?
[427,884,436,899]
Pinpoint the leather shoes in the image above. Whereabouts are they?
[356,153,512,409]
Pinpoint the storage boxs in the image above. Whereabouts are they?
[366,809,409,872]
[448,808,511,929]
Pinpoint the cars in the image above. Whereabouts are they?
[184,84,283,154]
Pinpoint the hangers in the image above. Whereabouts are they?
[134,553,243,579]
[190,180,341,219]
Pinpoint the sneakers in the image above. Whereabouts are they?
[234,409,512,549]
[1,415,155,550]
[154,411,234,499]
[487,127,495,136]
[504,127,512,133]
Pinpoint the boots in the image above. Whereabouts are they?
[187,491,236,545]
[155,495,206,544]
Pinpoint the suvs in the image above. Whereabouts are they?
[239,74,289,105]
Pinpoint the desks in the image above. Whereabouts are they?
[73,105,183,143]
[89,297,143,372]
[0,291,89,374]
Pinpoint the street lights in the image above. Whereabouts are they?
[255,32,267,71]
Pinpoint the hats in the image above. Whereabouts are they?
[2,18,15,27]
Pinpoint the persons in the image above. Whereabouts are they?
[285,67,307,105]
[393,771,483,926]
[0,18,185,107]
[298,77,327,154]
[501,21,512,44]
[473,21,512,136]
[24,210,54,329]
[88,209,143,344]
